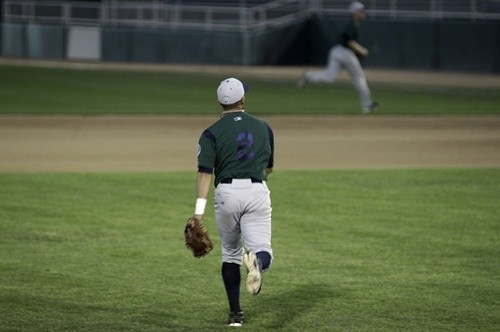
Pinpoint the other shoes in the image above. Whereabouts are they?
[243,250,264,295]
[298,69,311,89]
[362,102,380,114]
[227,312,245,327]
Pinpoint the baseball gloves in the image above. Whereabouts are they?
[183,215,215,260]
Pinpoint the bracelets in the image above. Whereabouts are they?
[195,198,206,215]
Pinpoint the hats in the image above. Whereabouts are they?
[349,2,365,13]
[217,78,248,105]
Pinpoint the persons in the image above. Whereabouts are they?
[298,2,381,113]
[195,77,275,327]
